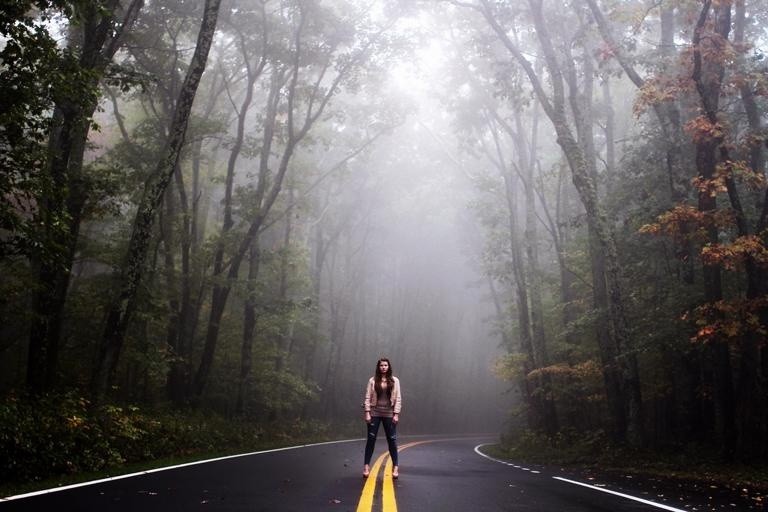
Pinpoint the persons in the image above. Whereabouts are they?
[360,356,403,479]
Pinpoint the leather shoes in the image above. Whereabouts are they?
[393,474,398,478]
[364,472,369,477]
